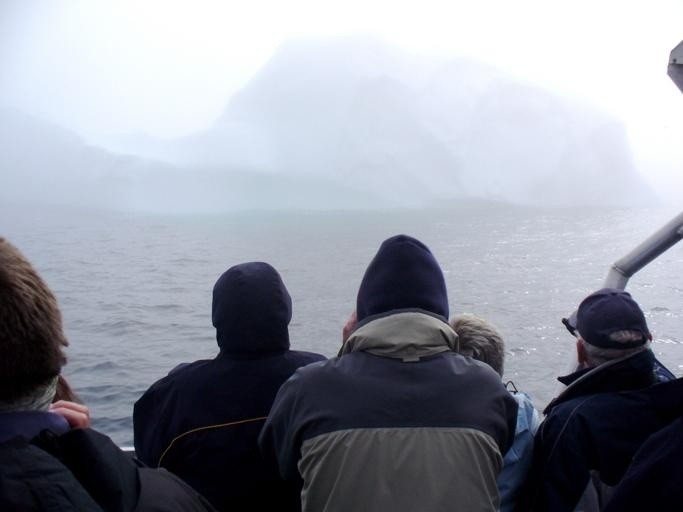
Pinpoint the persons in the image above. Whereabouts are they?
[1,234,220,512]
[134,236,683,512]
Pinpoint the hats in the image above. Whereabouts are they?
[356,233,450,321]
[575,287,652,349]
